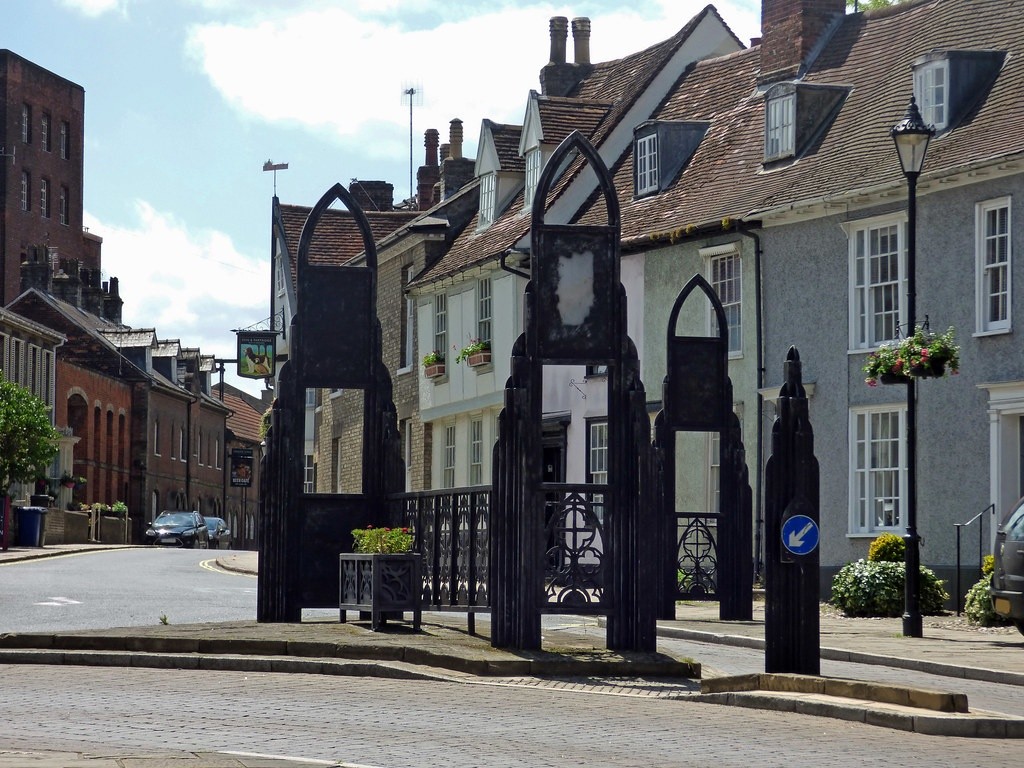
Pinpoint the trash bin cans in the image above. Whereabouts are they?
[13,506,49,548]
[29,495,55,547]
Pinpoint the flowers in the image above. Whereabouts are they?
[861,325,962,386]
[421,350,445,368]
[350,525,414,554]
[453,338,491,364]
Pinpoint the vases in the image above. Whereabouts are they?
[881,369,910,385]
[425,362,445,379]
[911,357,949,377]
[468,351,492,366]
[340,554,423,633]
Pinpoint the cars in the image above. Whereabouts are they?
[202,516,234,549]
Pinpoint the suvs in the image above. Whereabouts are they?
[145,510,209,549]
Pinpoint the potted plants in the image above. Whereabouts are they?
[90,501,126,516]
[60,470,88,490]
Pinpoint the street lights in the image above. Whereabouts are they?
[891,95,939,637]
[404,87,416,202]
